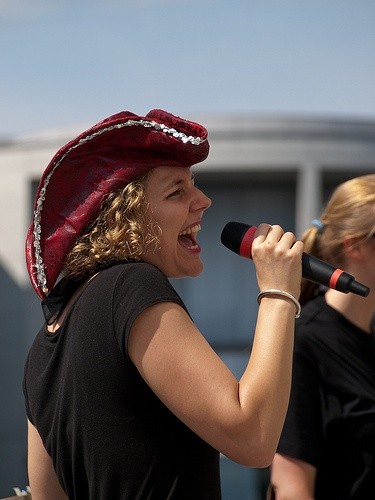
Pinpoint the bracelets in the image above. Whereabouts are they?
[257,288,302,318]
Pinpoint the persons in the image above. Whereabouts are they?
[22,109,302,500]
[270,173,374,499]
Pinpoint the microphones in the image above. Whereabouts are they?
[220,221,370,298]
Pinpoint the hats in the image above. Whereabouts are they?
[25,109,210,300]
